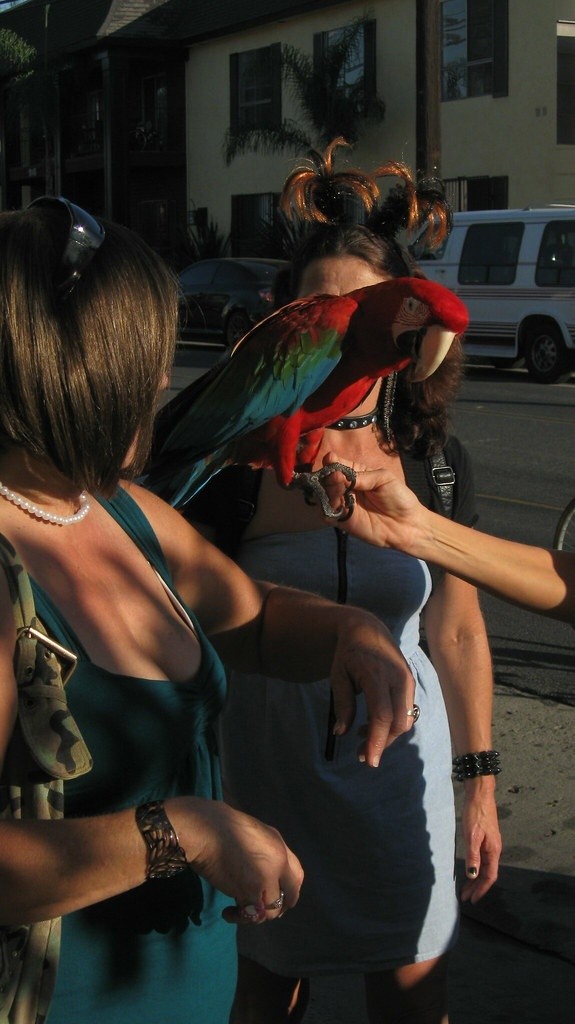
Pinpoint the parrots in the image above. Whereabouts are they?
[151,277,469,519]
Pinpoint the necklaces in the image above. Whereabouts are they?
[0,482,89,526]
[325,406,381,430]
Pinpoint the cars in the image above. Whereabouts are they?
[169,256,305,352]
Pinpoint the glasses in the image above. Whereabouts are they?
[26,194,107,298]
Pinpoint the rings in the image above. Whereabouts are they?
[407,703,419,725]
[262,888,288,909]
[238,904,266,925]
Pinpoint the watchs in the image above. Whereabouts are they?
[134,799,190,881]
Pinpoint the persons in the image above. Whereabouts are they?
[177,146,504,1024]
[0,208,417,1024]
[323,448,574,626]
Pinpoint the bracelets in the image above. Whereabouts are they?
[450,750,502,781]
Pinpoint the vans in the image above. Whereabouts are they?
[385,203,575,380]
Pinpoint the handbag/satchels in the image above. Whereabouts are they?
[0,532,94,1024]
